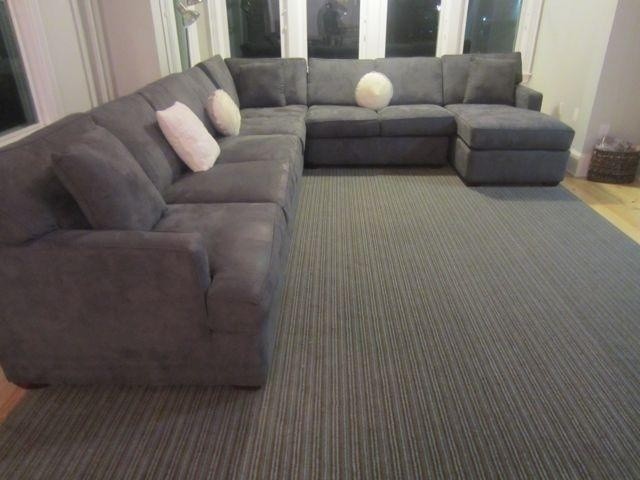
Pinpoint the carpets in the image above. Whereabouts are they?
[0,167,640,480]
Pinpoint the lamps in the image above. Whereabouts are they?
[174,0,206,28]
